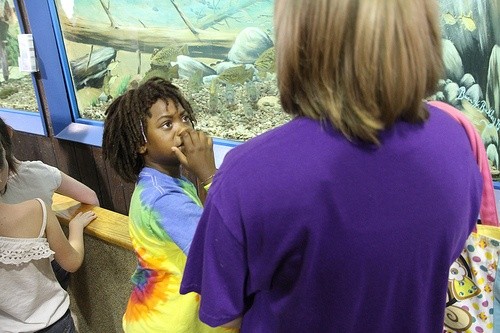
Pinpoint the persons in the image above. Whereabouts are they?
[101,76,241,333]
[0,140,98,333]
[1,116,100,293]
[178,0,481,333]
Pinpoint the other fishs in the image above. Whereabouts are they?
[217,65,253,84]
[176,0,271,32]
[145,64,180,83]
[254,47,276,78]
[240,89,253,119]
[462,15,477,32]
[224,81,236,107]
[187,69,204,91]
[245,78,259,103]
[209,78,218,96]
[149,44,190,66]
[443,13,456,25]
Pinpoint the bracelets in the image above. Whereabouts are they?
[200,172,215,185]
